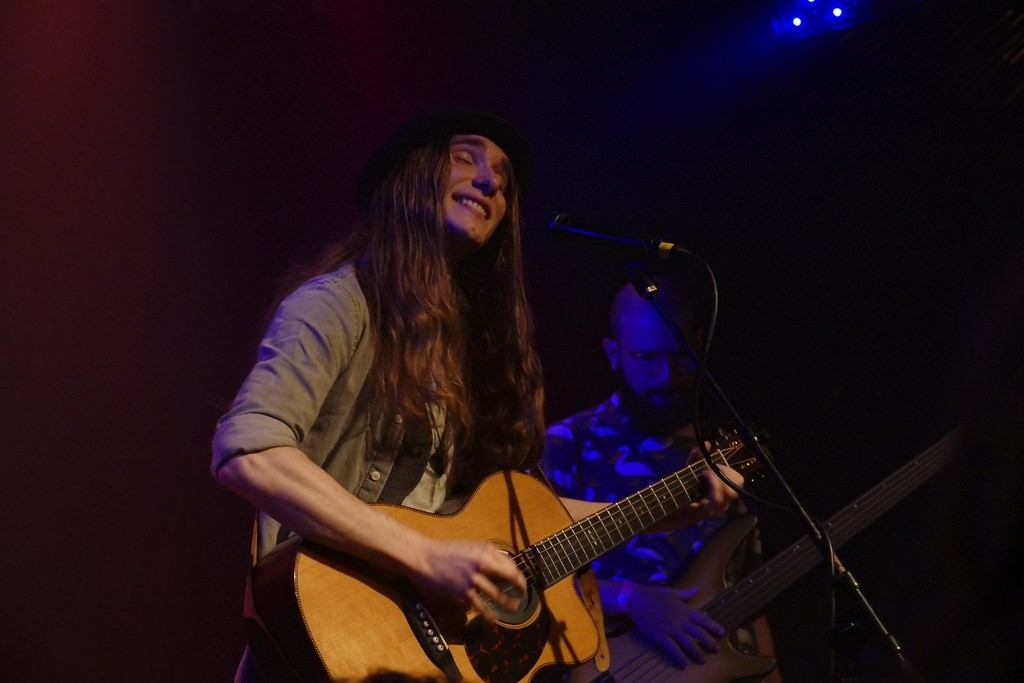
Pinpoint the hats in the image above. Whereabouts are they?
[356,106,539,209]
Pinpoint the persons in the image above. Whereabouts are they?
[205,103,746,682]
[522,277,787,683]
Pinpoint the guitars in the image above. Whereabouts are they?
[563,376,1016,682]
[254,419,777,681]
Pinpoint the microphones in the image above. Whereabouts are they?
[537,209,693,260]
